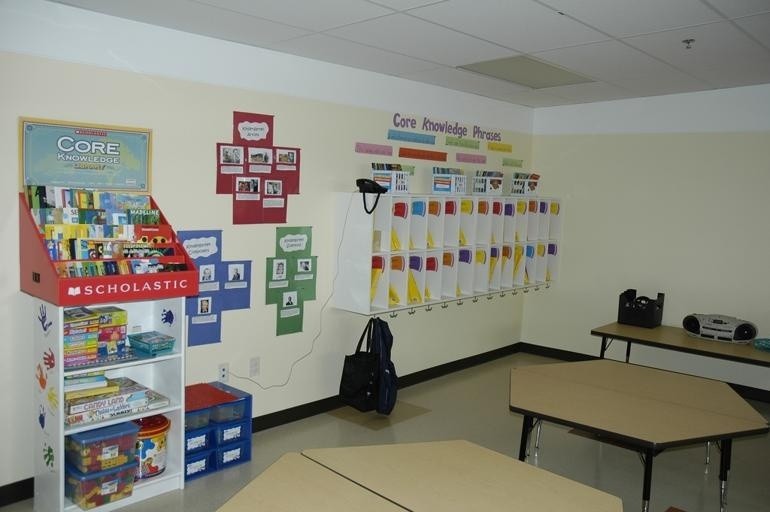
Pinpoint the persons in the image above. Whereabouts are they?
[275,263,283,275]
[286,295,294,305]
[223,147,295,194]
[231,268,241,281]
[202,269,212,281]
[301,262,312,272]
[200,299,209,313]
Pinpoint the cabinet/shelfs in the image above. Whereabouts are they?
[329,189,563,316]
[31,292,187,512]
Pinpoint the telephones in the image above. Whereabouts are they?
[357,178,387,193]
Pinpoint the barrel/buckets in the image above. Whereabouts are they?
[136,414,171,480]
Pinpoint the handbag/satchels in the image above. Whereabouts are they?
[339,351,399,415]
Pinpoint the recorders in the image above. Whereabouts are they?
[681,313,759,345]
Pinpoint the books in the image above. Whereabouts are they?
[25,184,190,426]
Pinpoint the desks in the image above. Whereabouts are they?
[502,358,768,511]
[215,438,627,512]
[588,323,769,377]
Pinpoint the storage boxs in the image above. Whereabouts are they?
[184,380,253,484]
